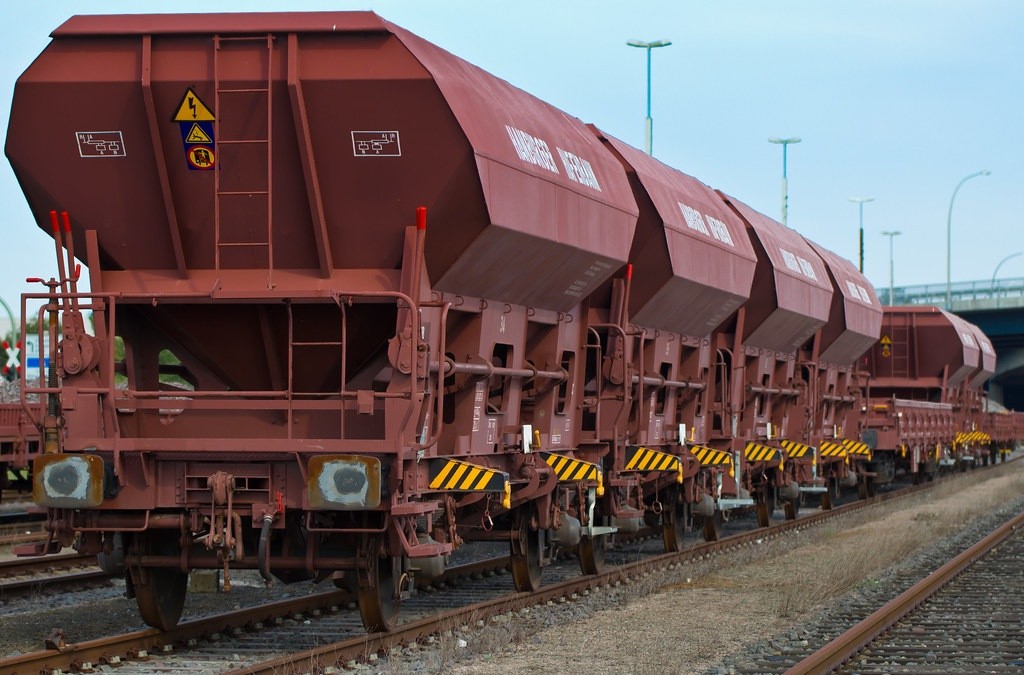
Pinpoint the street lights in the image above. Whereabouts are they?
[767,136,804,228]
[989,253,1019,336]
[946,168,986,314]
[848,195,876,276]
[624,34,673,153]
[881,228,902,309]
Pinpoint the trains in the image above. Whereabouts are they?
[0,400,105,548]
[11,12,1020,632]
